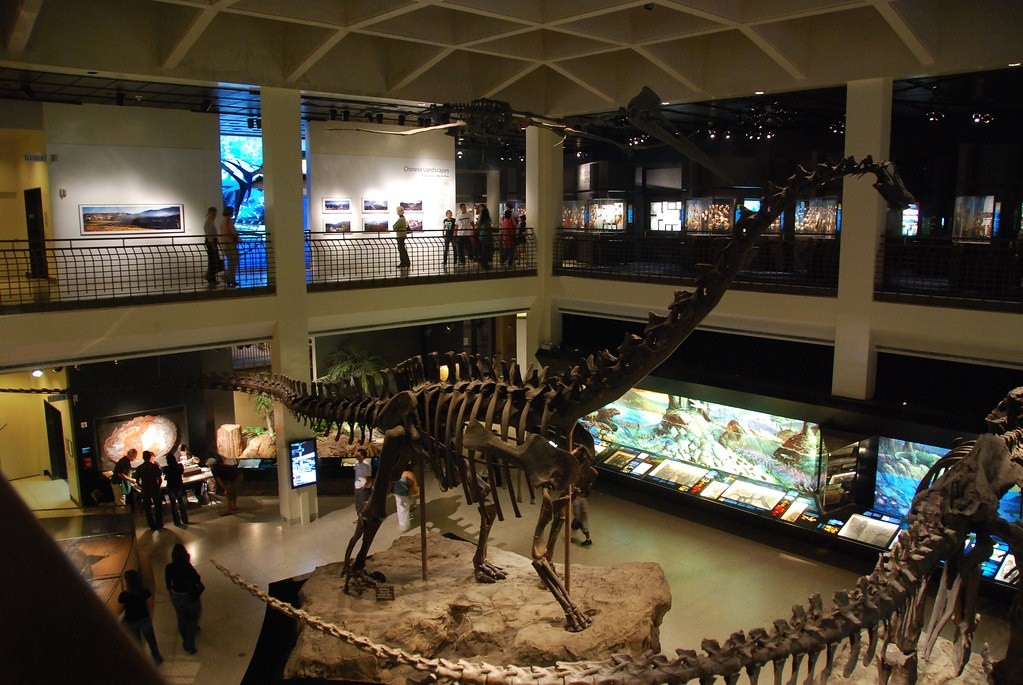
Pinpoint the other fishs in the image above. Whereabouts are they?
[220,151,307,224]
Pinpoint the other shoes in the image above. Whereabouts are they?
[220,510,233,516]
[581,539,591,545]
[227,280,240,286]
[352,520,358,525]
[397,263,410,268]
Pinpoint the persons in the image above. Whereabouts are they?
[117,569,164,667]
[499,202,526,266]
[395,463,417,534]
[220,207,242,285]
[618,215,622,223]
[393,207,410,267]
[353,449,372,524]
[111,449,137,513]
[136,451,164,530]
[164,544,205,655]
[441,210,457,264]
[205,458,243,516]
[162,453,188,525]
[454,203,475,265]
[571,493,592,546]
[178,444,204,502]
[476,204,494,269]
[564,216,572,226]
[204,207,223,289]
[592,210,598,220]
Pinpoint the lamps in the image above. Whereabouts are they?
[31,368,44,378]
[424,118,432,127]
[74,364,81,372]
[376,113,384,124]
[202,98,214,112]
[329,109,338,121]
[342,110,350,121]
[248,118,255,128]
[53,367,64,372]
[364,112,374,122]
[399,115,406,126]
[213,348,221,352]
[256,119,261,128]
[237,345,244,350]
[418,117,424,127]
[116,92,125,106]
[245,344,252,349]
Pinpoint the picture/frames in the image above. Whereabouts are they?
[322,196,425,234]
[78,203,186,235]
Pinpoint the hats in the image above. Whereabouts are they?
[519,215,526,219]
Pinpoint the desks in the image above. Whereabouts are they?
[130,467,213,518]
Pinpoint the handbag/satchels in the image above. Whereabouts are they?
[215,259,225,272]
[182,563,206,602]
[404,224,411,233]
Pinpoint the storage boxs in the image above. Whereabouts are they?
[183,467,202,477]
